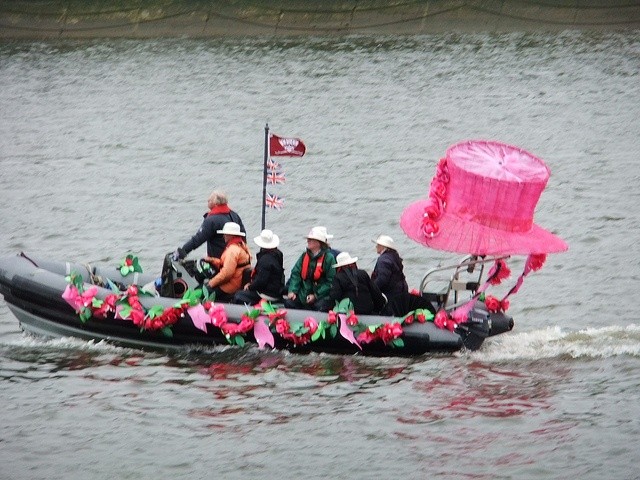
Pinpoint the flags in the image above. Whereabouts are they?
[266,159,286,185]
[265,192,287,211]
[268,131,306,157]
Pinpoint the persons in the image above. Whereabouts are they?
[172,192,247,275]
[371,234,405,316]
[285,226,336,312]
[231,229,285,306]
[334,251,378,315]
[200,222,252,303]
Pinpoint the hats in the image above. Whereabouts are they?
[332,252,358,269]
[254,230,279,249]
[372,235,398,250]
[314,226,333,240]
[303,229,325,242]
[217,222,245,236]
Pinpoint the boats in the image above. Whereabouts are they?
[0,244,514,358]
